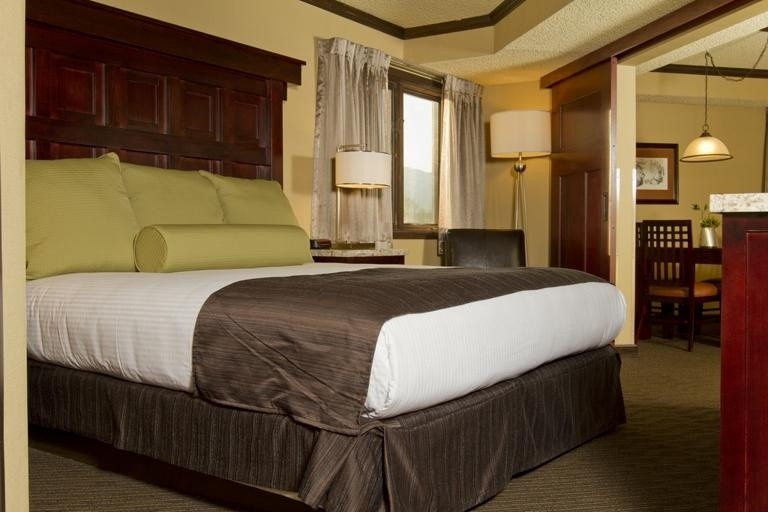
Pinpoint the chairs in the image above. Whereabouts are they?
[641,219,721,353]
[439,226,527,269]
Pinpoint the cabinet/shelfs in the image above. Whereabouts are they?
[709,193,766,512]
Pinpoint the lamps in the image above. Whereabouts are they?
[679,47,732,163]
[334,144,391,249]
[490,107,550,232]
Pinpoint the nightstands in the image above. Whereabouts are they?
[309,243,406,265]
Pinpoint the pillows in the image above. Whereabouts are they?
[202,171,316,264]
[24,148,141,282]
[133,222,311,269]
[117,155,223,231]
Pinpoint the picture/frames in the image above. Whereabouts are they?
[635,142,678,206]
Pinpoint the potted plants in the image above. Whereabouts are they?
[691,202,719,249]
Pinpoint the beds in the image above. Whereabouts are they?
[25,0,629,511]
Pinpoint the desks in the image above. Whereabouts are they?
[642,244,722,353]
